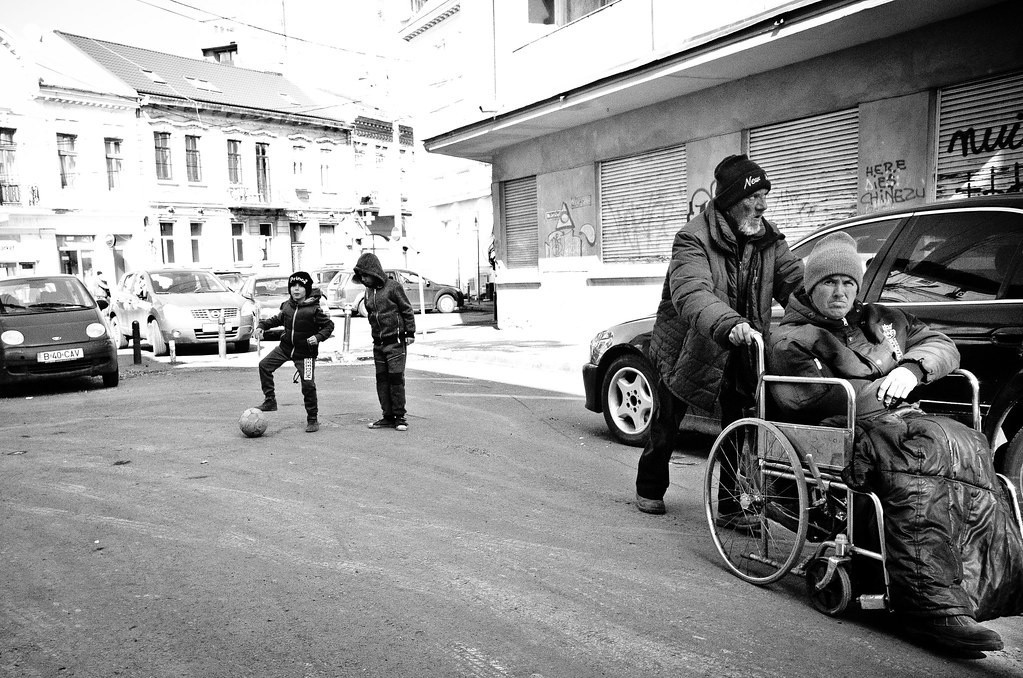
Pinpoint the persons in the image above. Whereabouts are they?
[95,271,111,317]
[256,272,334,433]
[769,231,1023,652]
[634,155,805,529]
[352,252,414,431]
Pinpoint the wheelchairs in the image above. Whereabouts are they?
[702,325,1022,620]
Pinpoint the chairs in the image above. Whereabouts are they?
[0,294,20,310]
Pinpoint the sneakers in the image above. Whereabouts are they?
[912,615,1004,651]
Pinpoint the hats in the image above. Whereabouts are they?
[714,154,771,211]
[288,271,313,298]
[804,231,863,296]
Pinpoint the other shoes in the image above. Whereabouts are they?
[306,421,319,432]
[254,401,277,411]
[396,421,407,430]
[368,418,395,428]
[636,493,665,513]
[716,511,767,530]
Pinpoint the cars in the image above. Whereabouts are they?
[240,275,331,334]
[0,273,120,388]
[580,193,1023,514]
[108,270,256,356]
[213,271,249,292]
[326,269,465,317]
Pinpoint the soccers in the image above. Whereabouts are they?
[237,407,268,437]
